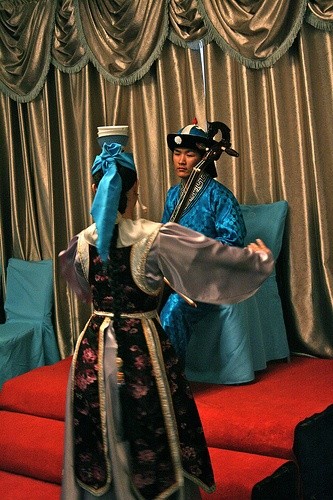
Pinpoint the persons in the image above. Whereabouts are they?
[56,126,275,500]
[155,116,269,385]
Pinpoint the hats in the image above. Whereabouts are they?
[167,115,223,161]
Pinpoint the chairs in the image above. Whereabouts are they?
[0,257,53,380]
[160,201,289,383]
